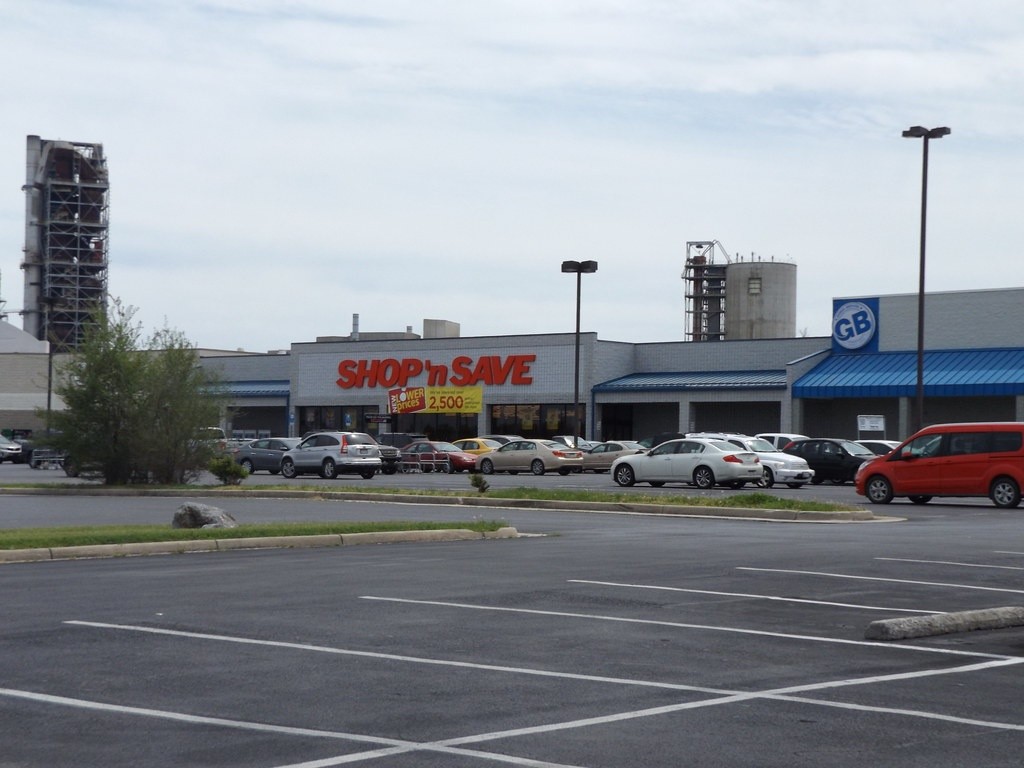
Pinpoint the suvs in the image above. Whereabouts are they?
[684,433,815,489]
[279,431,383,480]
[374,433,429,452]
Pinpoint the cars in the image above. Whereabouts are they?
[8,434,75,469]
[475,439,584,477]
[780,437,877,488]
[0,434,22,464]
[401,440,478,474]
[233,436,309,476]
[451,438,504,456]
[377,444,403,475]
[830,439,916,485]
[610,437,764,491]
[577,440,649,473]
[189,427,259,458]
[476,434,526,446]
[552,435,603,474]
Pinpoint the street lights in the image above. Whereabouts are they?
[901,125,952,431]
[561,260,598,446]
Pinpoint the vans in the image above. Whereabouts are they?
[854,421,1024,509]
[754,433,810,453]
